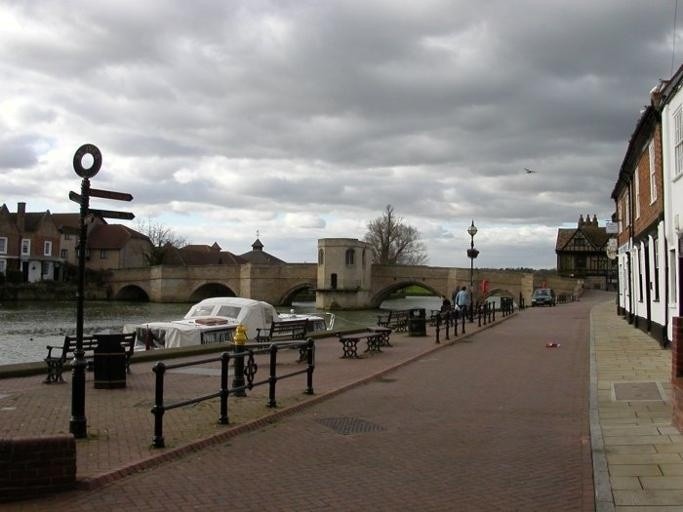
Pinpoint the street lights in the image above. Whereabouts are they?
[468,220,477,323]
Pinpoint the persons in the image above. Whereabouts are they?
[455,285,471,318]
[437,295,450,325]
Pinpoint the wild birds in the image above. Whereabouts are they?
[523,167,537,174]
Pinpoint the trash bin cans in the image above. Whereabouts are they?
[93,333,127,389]
[407,309,426,336]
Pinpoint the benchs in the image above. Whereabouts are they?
[339,333,382,359]
[255,318,309,353]
[377,310,410,333]
[367,326,392,346]
[43,331,138,384]
[429,309,453,327]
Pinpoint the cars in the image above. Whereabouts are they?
[531,288,556,307]
